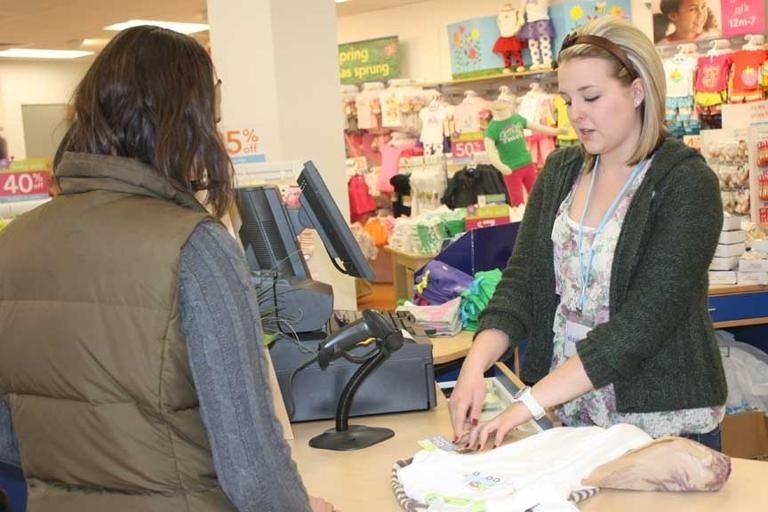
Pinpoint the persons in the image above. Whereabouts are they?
[443,12,728,454]
[484,102,569,208]
[653,0,723,45]
[0,26,337,512]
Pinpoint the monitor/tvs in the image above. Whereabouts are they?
[295,160,376,279]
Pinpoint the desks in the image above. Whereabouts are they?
[380,243,439,303]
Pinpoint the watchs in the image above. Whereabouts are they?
[511,385,546,422]
